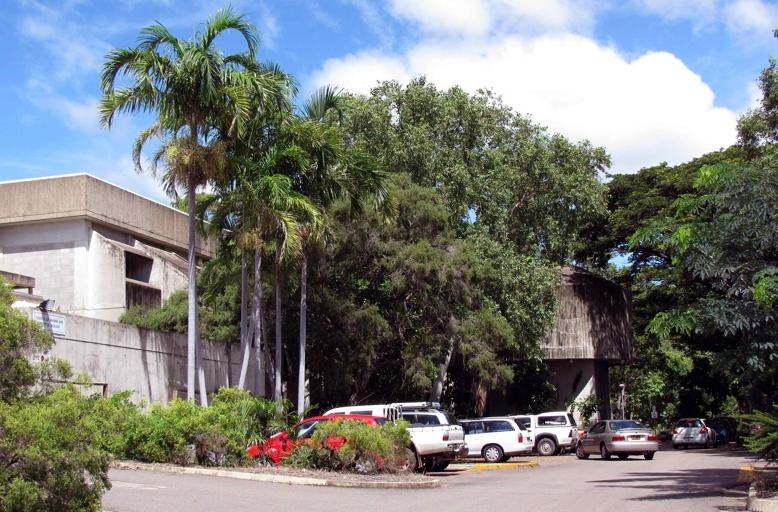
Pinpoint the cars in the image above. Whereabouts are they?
[245,413,396,475]
[672,417,719,448]
[457,417,534,463]
[576,419,659,459]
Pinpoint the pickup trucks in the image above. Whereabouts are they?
[508,411,578,456]
[296,404,466,475]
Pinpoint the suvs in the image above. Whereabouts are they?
[390,400,469,471]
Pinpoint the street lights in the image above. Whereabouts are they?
[619,383,625,419]
